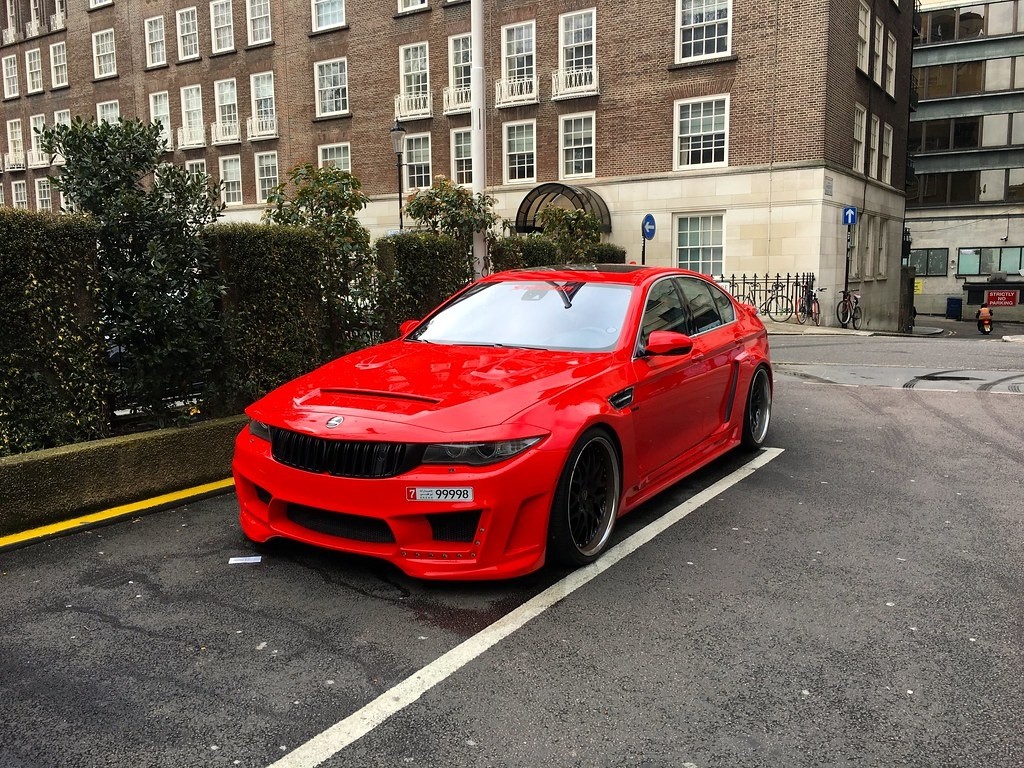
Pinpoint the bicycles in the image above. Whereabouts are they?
[732,284,793,323]
[794,282,827,327]
[836,288,862,330]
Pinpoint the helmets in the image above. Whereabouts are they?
[981,303,987,307]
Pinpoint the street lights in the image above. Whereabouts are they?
[387,116,409,233]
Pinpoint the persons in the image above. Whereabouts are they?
[975,302,993,329]
[913,306,917,326]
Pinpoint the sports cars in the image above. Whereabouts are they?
[231,262,774,585]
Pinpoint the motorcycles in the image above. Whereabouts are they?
[974,309,994,335]
